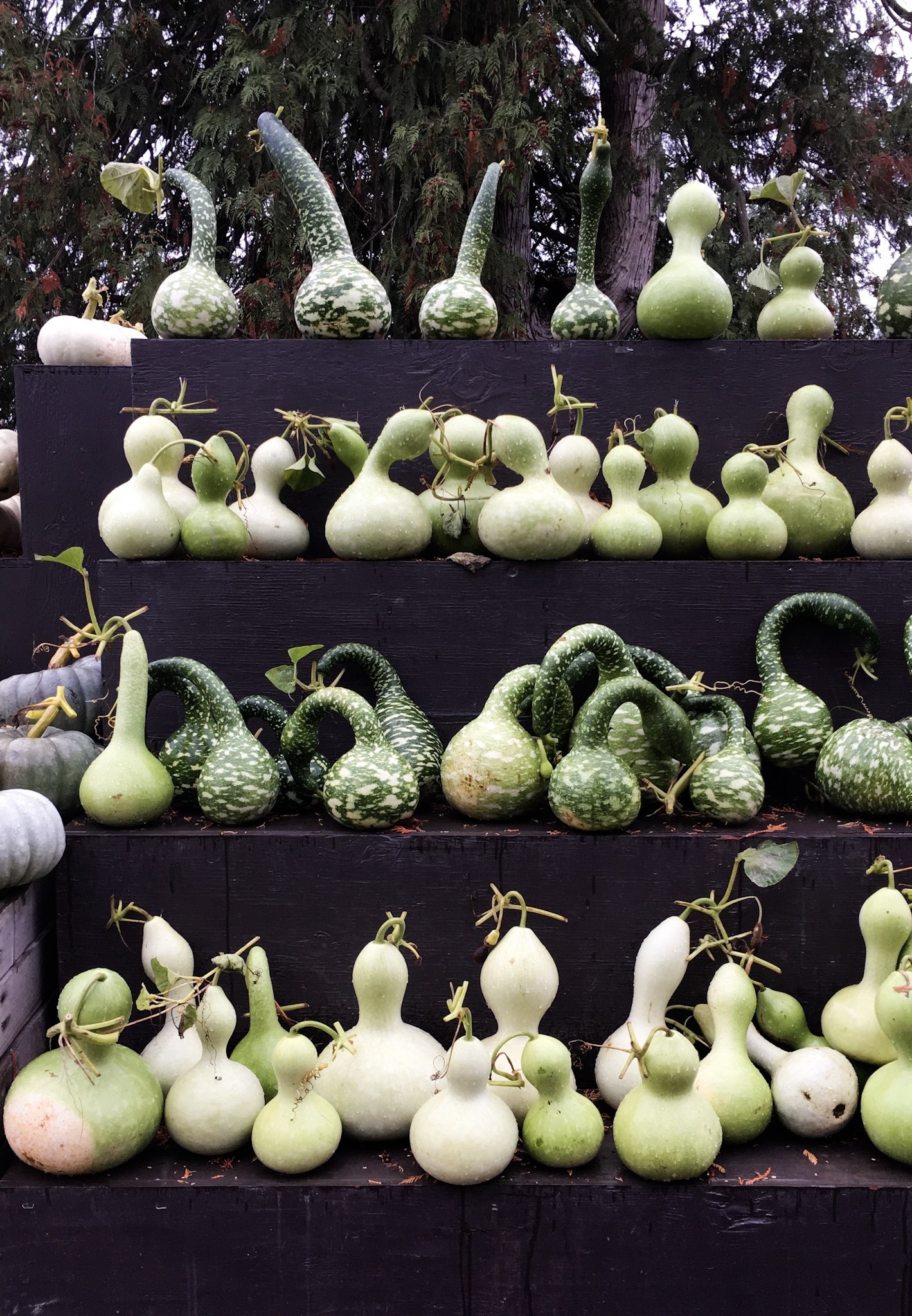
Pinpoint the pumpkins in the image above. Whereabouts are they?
[37,277,148,367]
[0,656,105,891]
[0,428,23,552]
